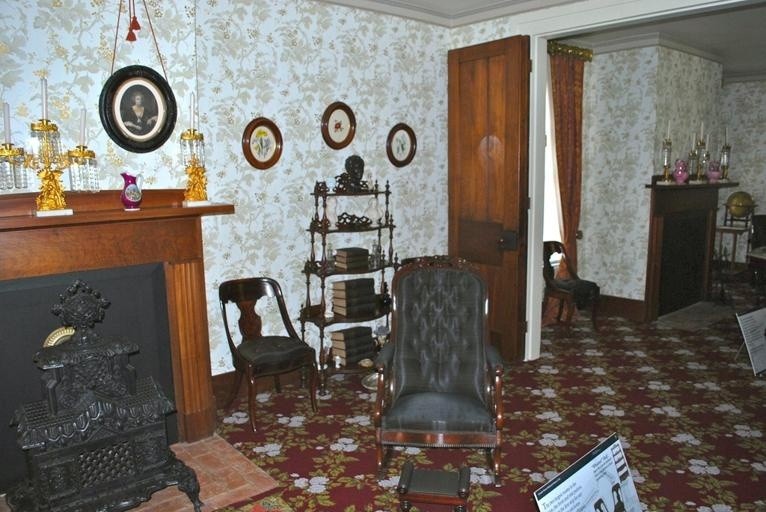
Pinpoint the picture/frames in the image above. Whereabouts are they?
[241,117,283,170]
[386,123,417,167]
[322,101,356,150]
[98,64,178,154]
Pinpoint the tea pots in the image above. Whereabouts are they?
[672,158,720,184]
[120,172,145,212]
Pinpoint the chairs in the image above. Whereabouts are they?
[375,253,506,488]
[543,241,601,337]
[218,276,320,435]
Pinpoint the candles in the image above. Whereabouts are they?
[725,127,729,146]
[667,119,670,142]
[41,76,48,120]
[190,92,195,130]
[80,107,85,146]
[3,102,11,144]
[691,120,710,153]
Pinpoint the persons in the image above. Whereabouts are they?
[123,90,158,133]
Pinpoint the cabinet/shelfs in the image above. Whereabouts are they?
[297,181,400,376]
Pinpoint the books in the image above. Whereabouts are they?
[530,431,643,512]
[329,247,377,367]
[735,303,766,377]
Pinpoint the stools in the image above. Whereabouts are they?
[397,461,471,512]
[713,225,751,284]
